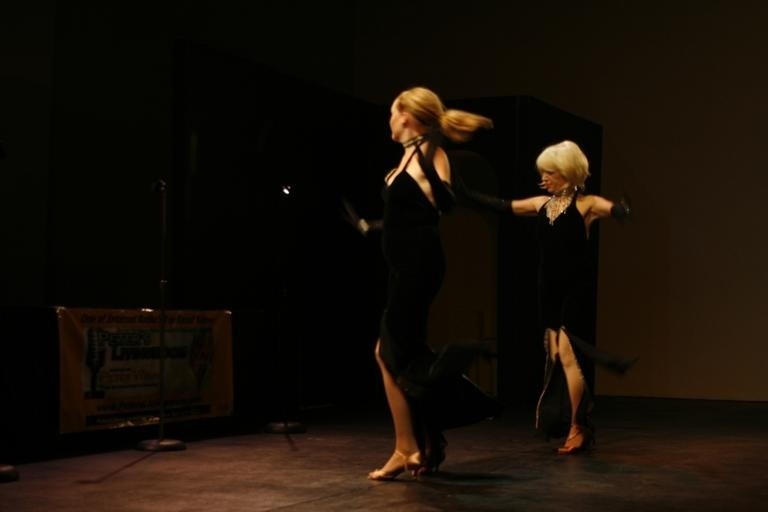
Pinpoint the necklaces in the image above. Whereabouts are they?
[402,134,427,149]
[546,185,578,226]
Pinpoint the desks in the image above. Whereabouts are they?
[9,304,235,458]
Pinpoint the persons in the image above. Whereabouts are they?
[462,139,632,455]
[338,86,492,483]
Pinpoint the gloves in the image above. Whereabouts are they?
[341,200,384,233]
[610,192,633,221]
[456,178,514,221]
[412,139,457,218]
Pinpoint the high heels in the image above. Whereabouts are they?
[551,420,596,457]
[369,450,446,481]
